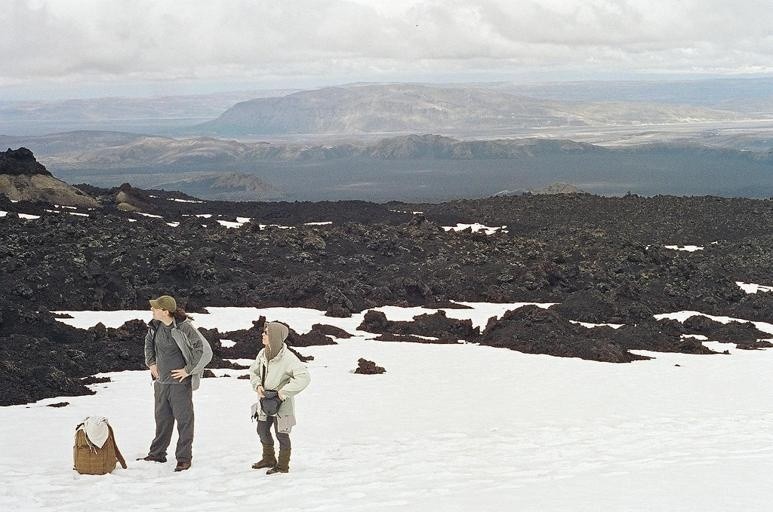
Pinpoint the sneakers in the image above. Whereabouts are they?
[137,455,166,463]
[174,461,191,471]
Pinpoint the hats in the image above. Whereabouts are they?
[149,295,177,313]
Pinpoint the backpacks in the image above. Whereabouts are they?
[73,418,126,475]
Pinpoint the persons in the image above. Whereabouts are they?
[249,321,311,475]
[135,295,213,471]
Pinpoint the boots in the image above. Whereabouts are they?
[253,445,290,474]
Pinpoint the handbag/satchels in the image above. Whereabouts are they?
[260,389,282,416]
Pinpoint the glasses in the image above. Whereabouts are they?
[263,330,268,336]
